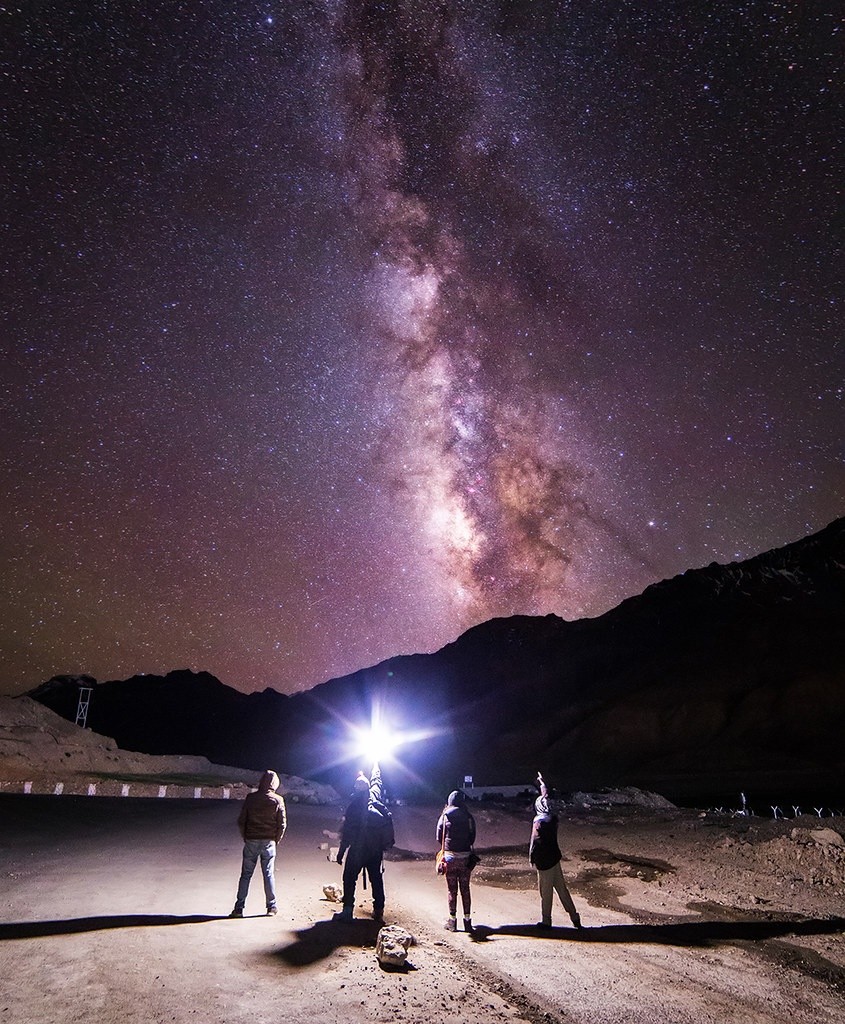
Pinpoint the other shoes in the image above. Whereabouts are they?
[537,922,551,929]
[573,920,581,927]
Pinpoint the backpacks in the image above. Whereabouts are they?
[349,802,384,890]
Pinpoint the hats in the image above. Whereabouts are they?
[535,796,554,815]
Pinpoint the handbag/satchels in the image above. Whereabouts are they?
[436,850,447,874]
[468,854,481,871]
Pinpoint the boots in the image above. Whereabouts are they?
[333,904,354,922]
[444,917,456,931]
[463,919,474,931]
[371,901,384,922]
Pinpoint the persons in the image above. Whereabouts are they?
[228,769,287,917]
[435,790,476,933]
[529,771,583,931]
[333,777,394,925]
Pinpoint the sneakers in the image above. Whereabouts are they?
[266,907,278,916]
[230,910,243,917]
